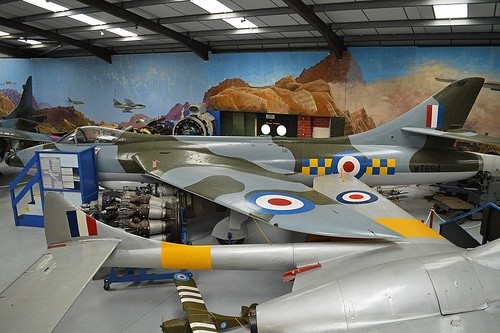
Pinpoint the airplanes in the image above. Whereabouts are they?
[0,75,500,244]
[0,174,499,333]
